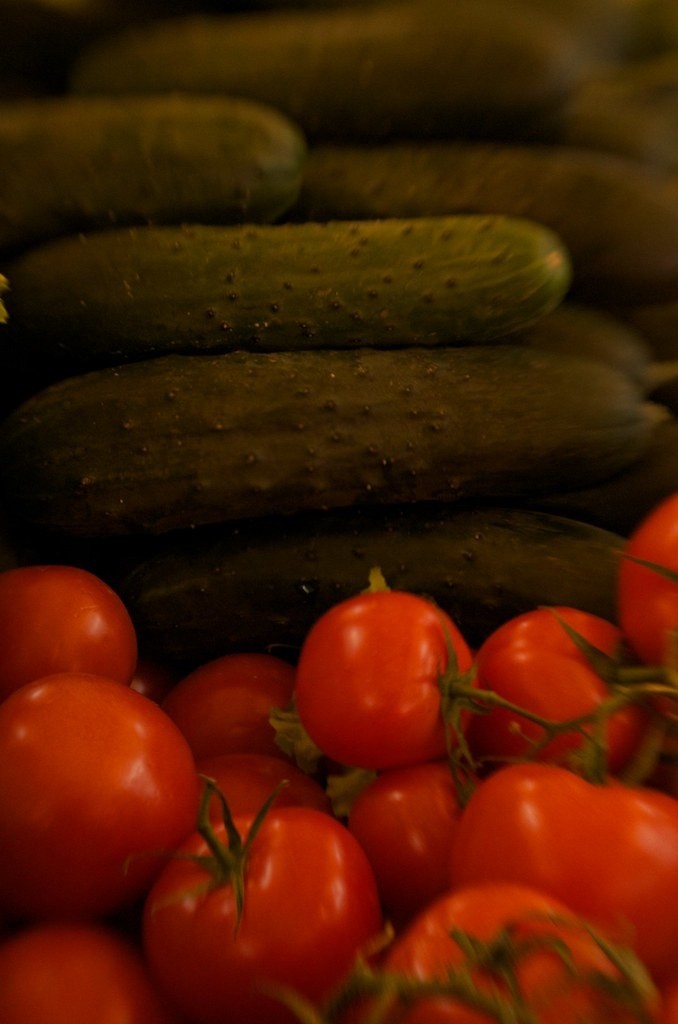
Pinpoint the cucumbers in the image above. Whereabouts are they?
[1,1,678,648]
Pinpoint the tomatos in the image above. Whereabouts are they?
[0,496,678,1024]
